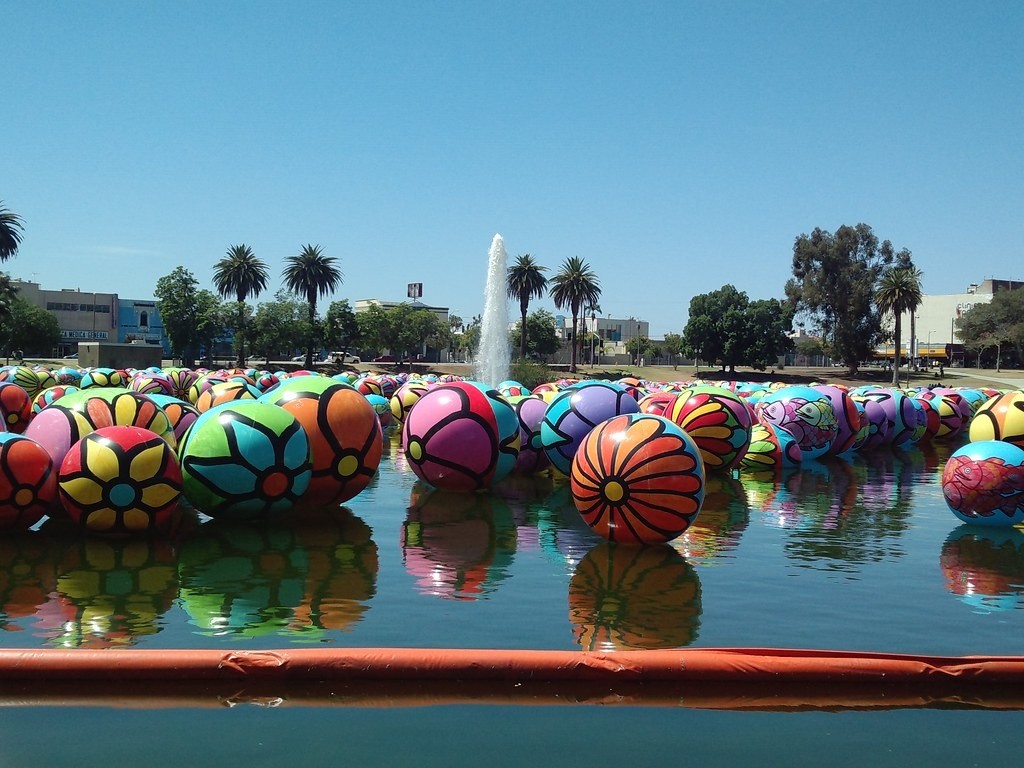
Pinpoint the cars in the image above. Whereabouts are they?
[373,356,399,362]
[291,354,317,362]
[63,353,78,359]
[402,356,428,362]
[244,354,267,361]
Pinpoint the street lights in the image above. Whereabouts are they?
[913,316,920,367]
[927,329,937,367]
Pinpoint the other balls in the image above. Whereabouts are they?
[1,445,1018,649]
[0,364,1024,545]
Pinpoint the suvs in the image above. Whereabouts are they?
[327,352,361,364]
